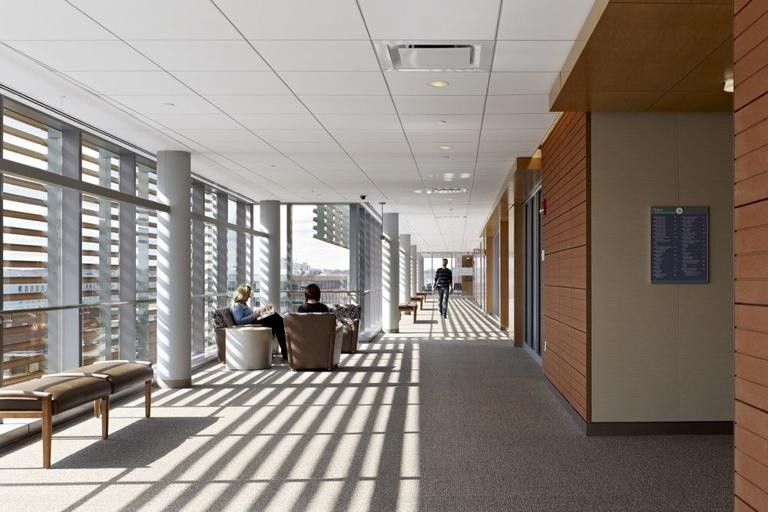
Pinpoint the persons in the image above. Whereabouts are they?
[430,258,454,320]
[296,282,329,313]
[228,282,288,360]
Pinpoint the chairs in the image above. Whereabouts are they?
[209,303,362,372]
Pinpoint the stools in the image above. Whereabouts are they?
[398,292,426,323]
[0,360,154,469]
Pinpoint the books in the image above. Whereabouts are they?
[253,306,276,320]
